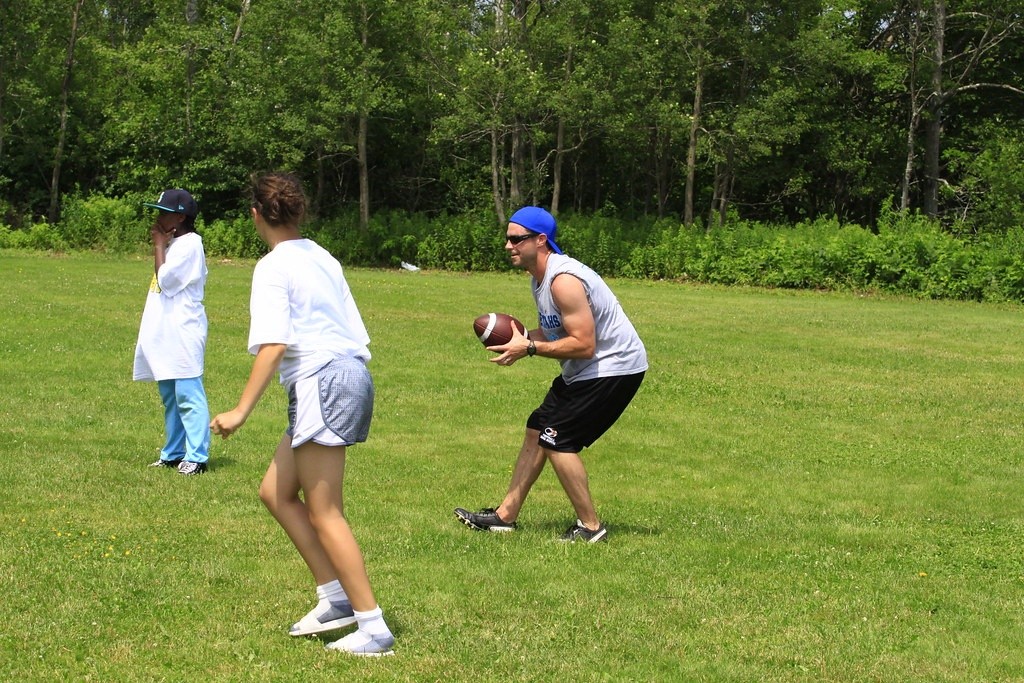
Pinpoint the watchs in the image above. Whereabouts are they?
[526,340,537,357]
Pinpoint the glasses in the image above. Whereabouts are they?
[506,233,539,244]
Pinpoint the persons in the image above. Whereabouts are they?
[209,173,398,659]
[131,187,214,475]
[452,205,648,546]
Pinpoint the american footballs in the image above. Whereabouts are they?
[473,313,531,354]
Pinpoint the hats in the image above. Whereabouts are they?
[508,206,562,255]
[142,188,199,218]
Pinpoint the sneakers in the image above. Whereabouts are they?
[453,506,517,531]
[561,518,607,543]
[177,460,207,475]
[148,460,181,468]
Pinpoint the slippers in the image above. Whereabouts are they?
[323,631,395,657]
[288,607,356,636]
